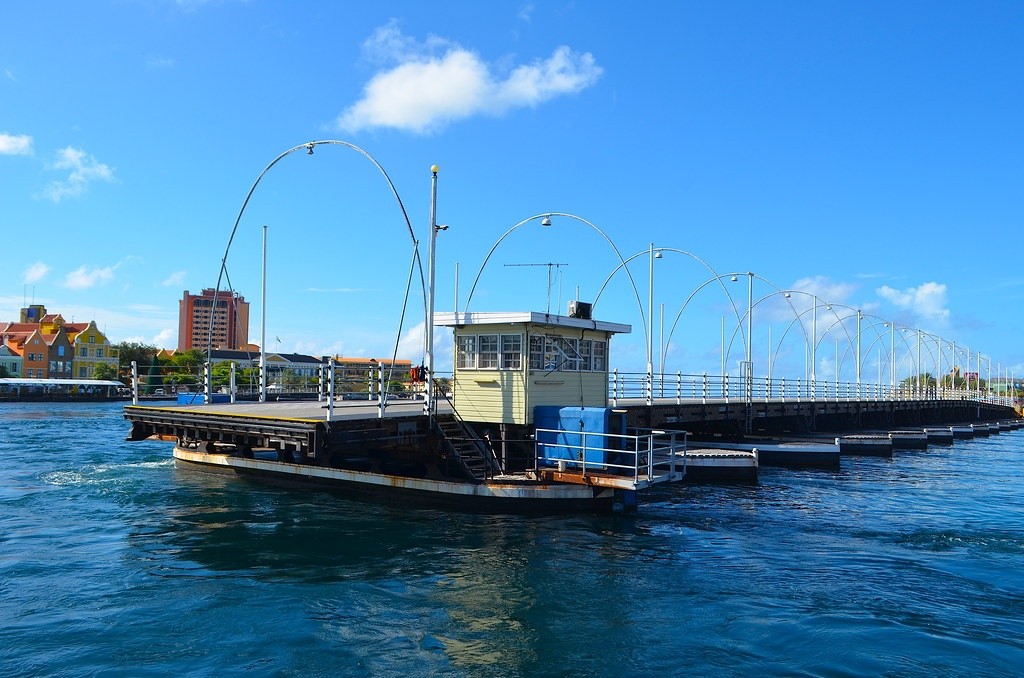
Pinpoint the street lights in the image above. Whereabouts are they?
[425,164,439,413]
